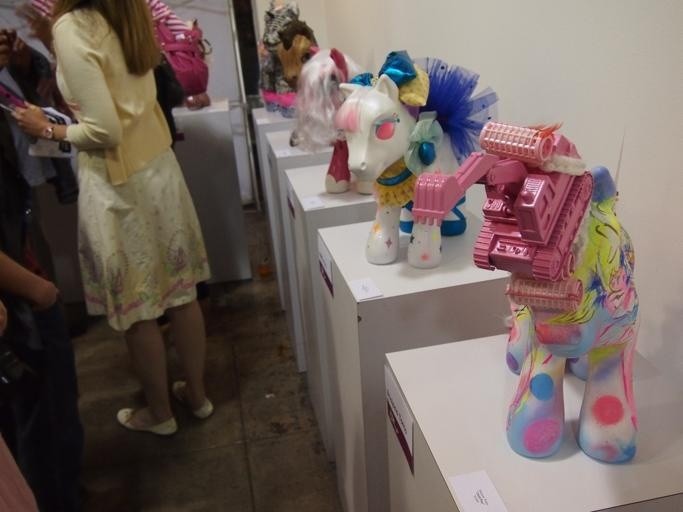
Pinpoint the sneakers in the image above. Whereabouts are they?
[172,379,213,416]
[116,407,176,435]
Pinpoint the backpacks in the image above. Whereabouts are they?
[143,1,209,93]
